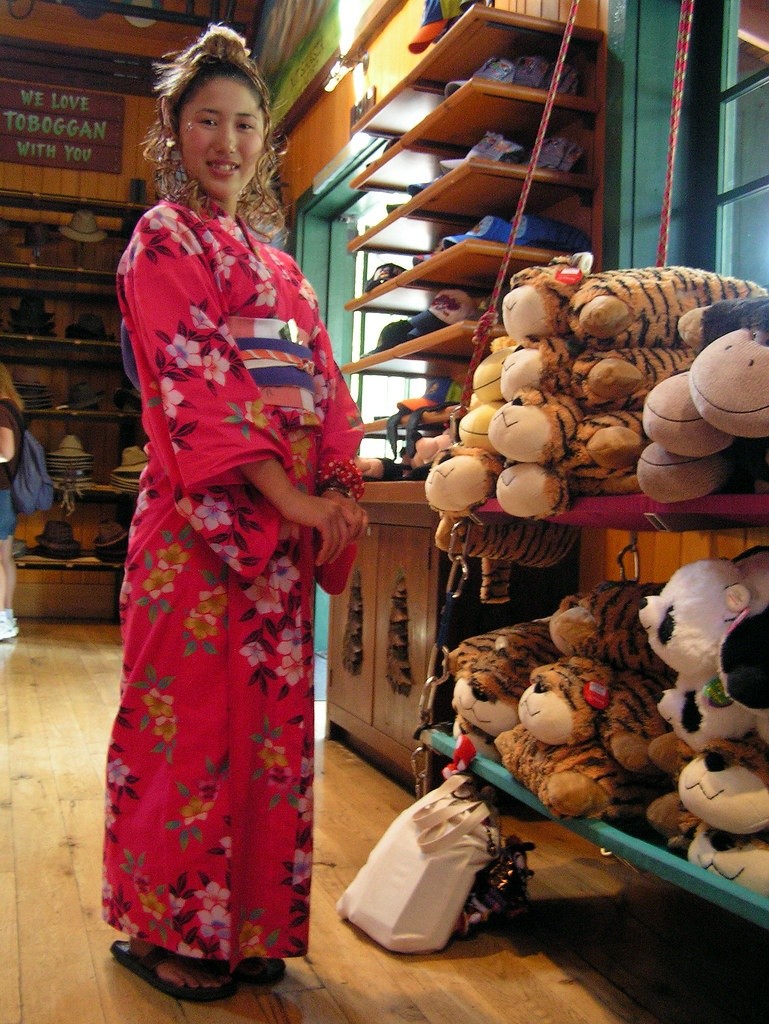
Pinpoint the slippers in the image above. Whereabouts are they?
[110,941,286,1002]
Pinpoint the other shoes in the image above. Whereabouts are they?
[0,616,20,640]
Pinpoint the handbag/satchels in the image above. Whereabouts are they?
[336,774,500,954]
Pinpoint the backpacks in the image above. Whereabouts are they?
[0,400,53,516]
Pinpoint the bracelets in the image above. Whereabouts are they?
[317,480,351,498]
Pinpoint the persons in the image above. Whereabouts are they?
[102,22,372,1003]
[0,362,24,640]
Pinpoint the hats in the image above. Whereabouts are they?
[442,216,512,244]
[6,295,149,564]
[408,173,445,196]
[408,288,474,336]
[397,378,462,412]
[17,222,62,248]
[60,209,108,242]
[439,134,525,164]
[526,137,585,172]
[510,214,590,253]
[412,242,444,266]
[366,138,400,168]
[387,204,405,214]
[445,56,585,96]
[360,319,413,360]
[408,0,475,54]
[12,540,27,558]
[365,263,407,291]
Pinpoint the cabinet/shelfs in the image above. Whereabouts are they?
[421,483,769,929]
[323,8,608,800]
[0,188,160,620]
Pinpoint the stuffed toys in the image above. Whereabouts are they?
[353,253,769,900]
